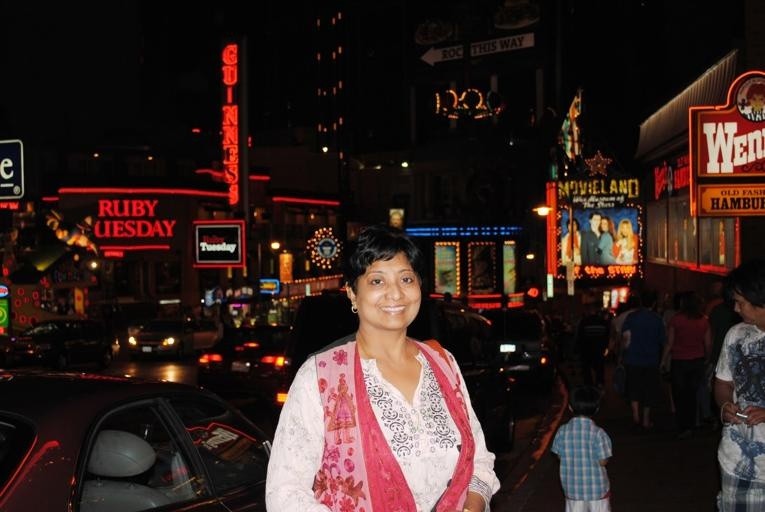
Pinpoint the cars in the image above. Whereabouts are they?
[1,364,274,511]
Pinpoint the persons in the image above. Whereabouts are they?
[560,210,638,267]
[265,222,503,511]
[551,288,765,512]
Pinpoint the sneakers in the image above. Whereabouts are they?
[632,423,655,435]
[671,418,722,437]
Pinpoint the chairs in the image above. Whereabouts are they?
[79,411,203,512]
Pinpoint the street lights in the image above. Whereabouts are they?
[531,181,578,296]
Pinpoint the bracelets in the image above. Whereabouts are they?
[463,508,469,512]
